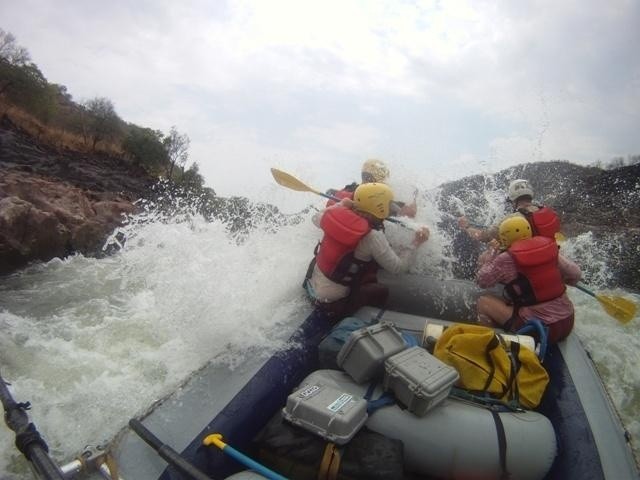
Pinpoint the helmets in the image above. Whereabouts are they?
[508,179,534,201]
[499,216,532,246]
[363,158,389,183]
[353,182,394,219]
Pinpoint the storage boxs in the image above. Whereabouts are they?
[381,345,461,419]
[333,319,412,385]
[281,381,371,448]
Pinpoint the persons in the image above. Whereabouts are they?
[325,155,420,220]
[459,176,562,257]
[304,182,431,321]
[474,216,583,344]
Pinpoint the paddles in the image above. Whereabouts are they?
[269,169,417,232]
[577,285,636,323]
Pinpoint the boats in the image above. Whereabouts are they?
[35,203,640,480]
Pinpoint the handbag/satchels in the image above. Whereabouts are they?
[433,323,551,409]
